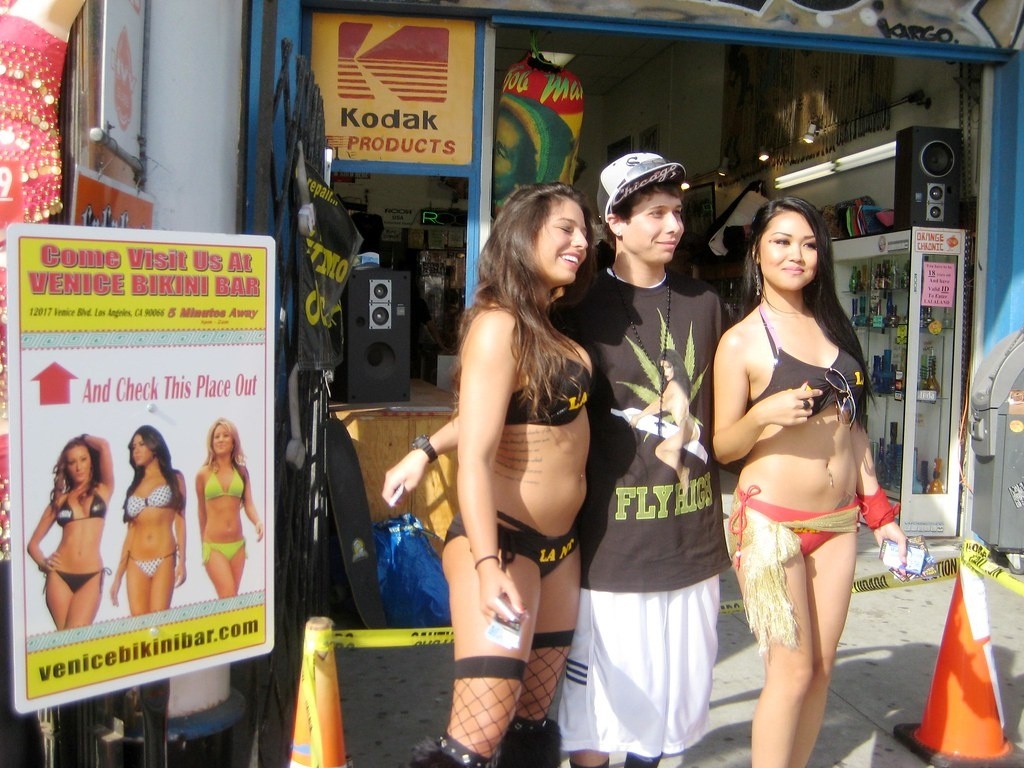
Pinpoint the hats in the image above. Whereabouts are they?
[599,153,686,229]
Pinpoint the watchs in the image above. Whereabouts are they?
[411,434,438,464]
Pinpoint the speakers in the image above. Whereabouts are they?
[892,127,965,232]
[328,269,411,403]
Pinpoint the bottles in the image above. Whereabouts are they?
[849,259,909,291]
[871,348,939,396]
[871,422,942,494]
[850,296,951,328]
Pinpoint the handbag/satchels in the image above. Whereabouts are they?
[702,178,769,263]
[490,51,584,221]
[372,513,452,628]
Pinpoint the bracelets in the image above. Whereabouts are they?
[857,483,900,532]
[474,556,500,570]
[38,558,48,571]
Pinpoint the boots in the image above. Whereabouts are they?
[406,739,464,768]
[570,758,609,768]
[496,713,561,768]
[624,752,665,768]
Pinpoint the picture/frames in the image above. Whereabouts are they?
[681,181,716,241]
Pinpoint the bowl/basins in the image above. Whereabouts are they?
[875,211,894,227]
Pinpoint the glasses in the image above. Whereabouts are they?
[824,366,856,429]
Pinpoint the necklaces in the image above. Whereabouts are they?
[611,266,671,442]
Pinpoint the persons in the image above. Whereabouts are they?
[110,426,186,616]
[196,418,263,600]
[0,0,85,224]
[408,180,593,768]
[382,154,736,768]
[27,434,114,632]
[713,196,909,768]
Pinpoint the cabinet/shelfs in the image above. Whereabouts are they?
[825,227,965,538]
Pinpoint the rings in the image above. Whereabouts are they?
[48,559,52,564]
[802,401,810,410]
[493,612,499,622]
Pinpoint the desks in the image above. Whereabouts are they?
[327,378,459,558]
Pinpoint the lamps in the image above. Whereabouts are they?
[758,146,769,160]
[803,123,817,143]
[775,141,896,192]
[718,158,728,176]
